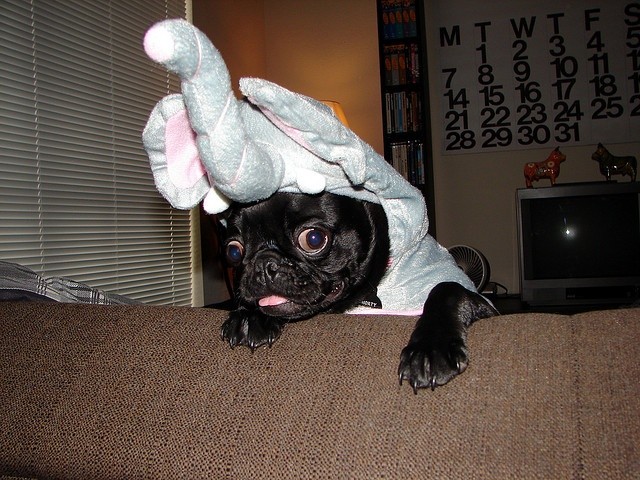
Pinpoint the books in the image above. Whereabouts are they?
[382,41,419,86]
[384,88,421,133]
[390,137,426,187]
[381,1,417,40]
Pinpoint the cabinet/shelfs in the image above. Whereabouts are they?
[485,292,638,324]
[377,2,438,239]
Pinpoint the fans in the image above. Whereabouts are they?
[442,245,491,294]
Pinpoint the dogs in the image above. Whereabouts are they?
[218,187,504,397]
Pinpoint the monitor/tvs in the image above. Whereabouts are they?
[514,182,639,309]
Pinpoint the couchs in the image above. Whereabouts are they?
[1,294,638,478]
